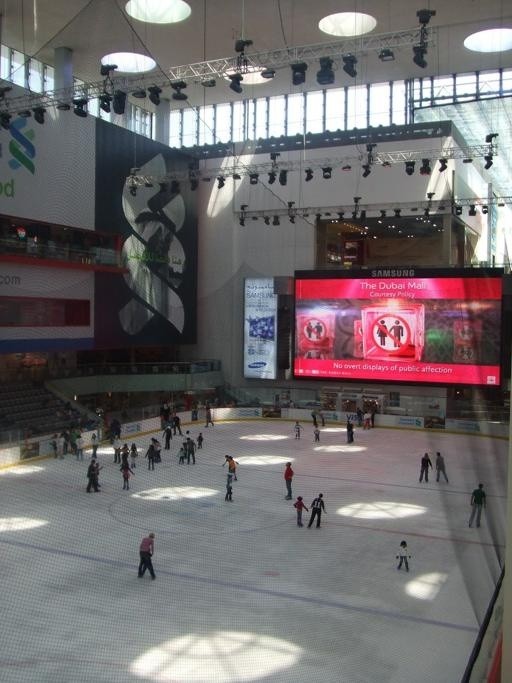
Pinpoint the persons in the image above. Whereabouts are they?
[307,493,326,528]
[138,533,155,579]
[396,541,411,571]
[346,400,376,443]
[468,484,486,527]
[48,398,238,501]
[289,400,294,409]
[141,548,152,574]
[419,453,432,482]
[294,421,303,439]
[436,452,448,482]
[294,497,308,528]
[284,462,293,499]
[311,409,324,441]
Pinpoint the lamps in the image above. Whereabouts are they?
[0,9,499,226]
